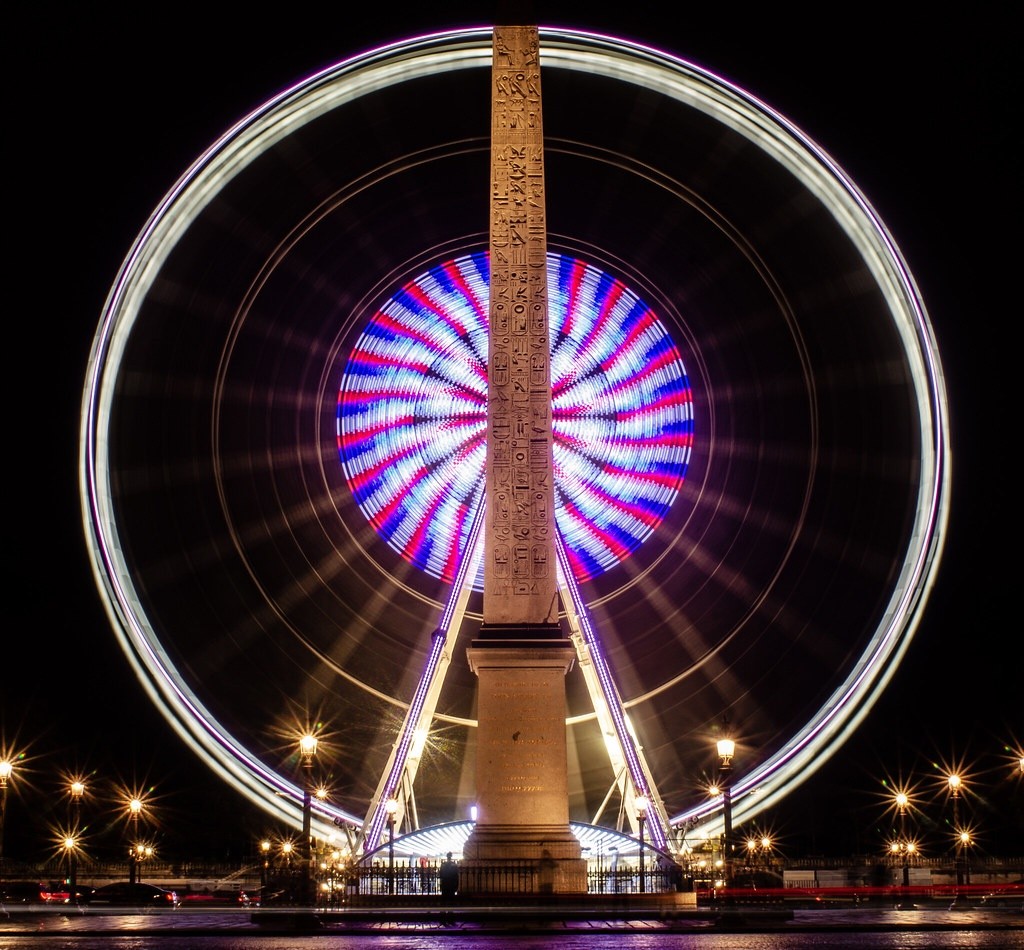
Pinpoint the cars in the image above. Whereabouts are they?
[722,870,784,888]
[213,889,250,909]
[0,880,70,907]
[75,882,179,909]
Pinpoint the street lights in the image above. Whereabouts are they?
[385,799,397,896]
[70,780,85,905]
[128,846,155,882]
[949,773,962,885]
[959,831,971,885]
[716,738,734,887]
[635,797,648,893]
[298,727,319,906]
[261,839,292,905]
[897,793,910,885]
[126,798,140,891]
[749,840,771,870]
[0,757,13,868]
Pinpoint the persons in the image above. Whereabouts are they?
[439,851,460,926]
